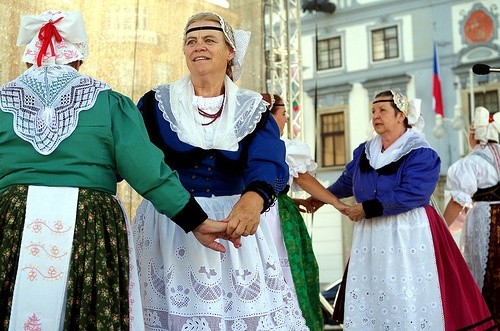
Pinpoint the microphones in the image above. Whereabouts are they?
[472,64,500,75]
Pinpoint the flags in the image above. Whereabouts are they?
[431,42,444,137]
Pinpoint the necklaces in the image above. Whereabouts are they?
[197,86,225,125]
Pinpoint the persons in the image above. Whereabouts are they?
[0,9,240,331]
[132,11,309,331]
[259,93,353,331]
[442,107,500,328]
[305,91,494,331]
